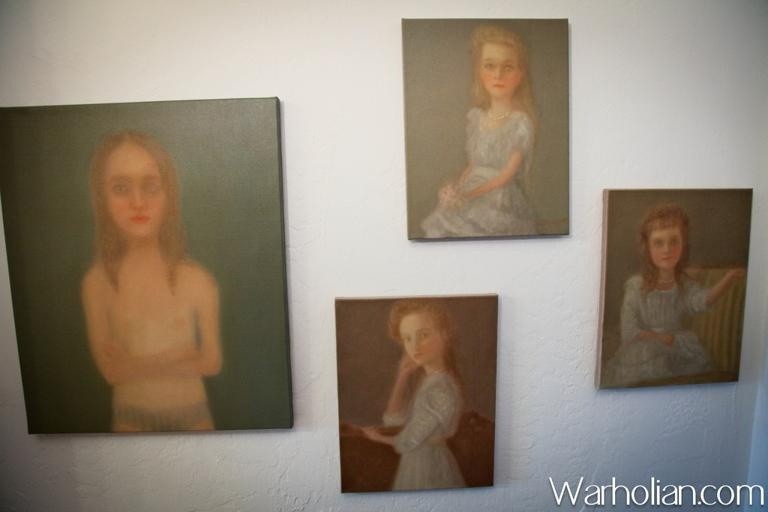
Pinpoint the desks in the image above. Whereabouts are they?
[340,410,495,492]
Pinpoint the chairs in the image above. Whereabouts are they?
[627,266,745,389]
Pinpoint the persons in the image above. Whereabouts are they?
[349,300,466,489]
[418,26,547,241]
[81,131,222,431]
[605,202,746,388]
